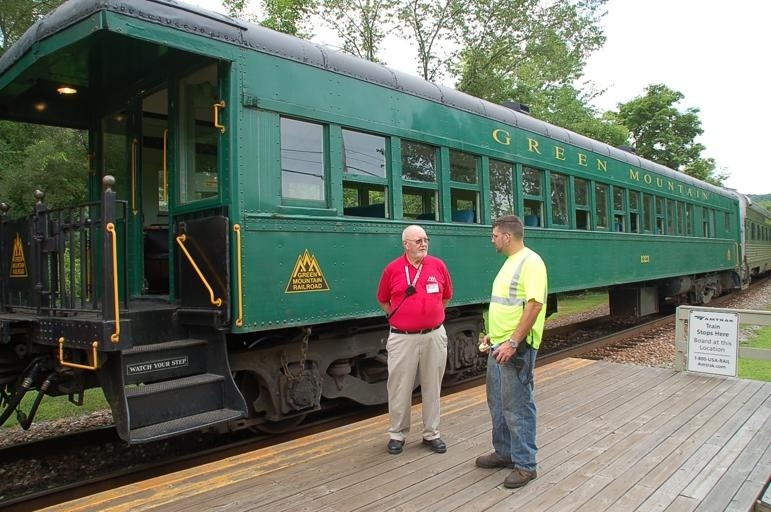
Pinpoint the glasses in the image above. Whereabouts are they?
[407,238,430,244]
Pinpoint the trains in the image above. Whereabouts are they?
[0,0,771,445]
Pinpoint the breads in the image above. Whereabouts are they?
[478,343,489,352]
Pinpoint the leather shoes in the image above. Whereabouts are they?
[476,451,514,468]
[504,468,537,488]
[386,437,405,454]
[423,437,446,453]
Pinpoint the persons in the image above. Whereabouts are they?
[377,224,453,454]
[476,215,548,488]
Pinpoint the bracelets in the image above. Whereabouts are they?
[510,339,518,348]
